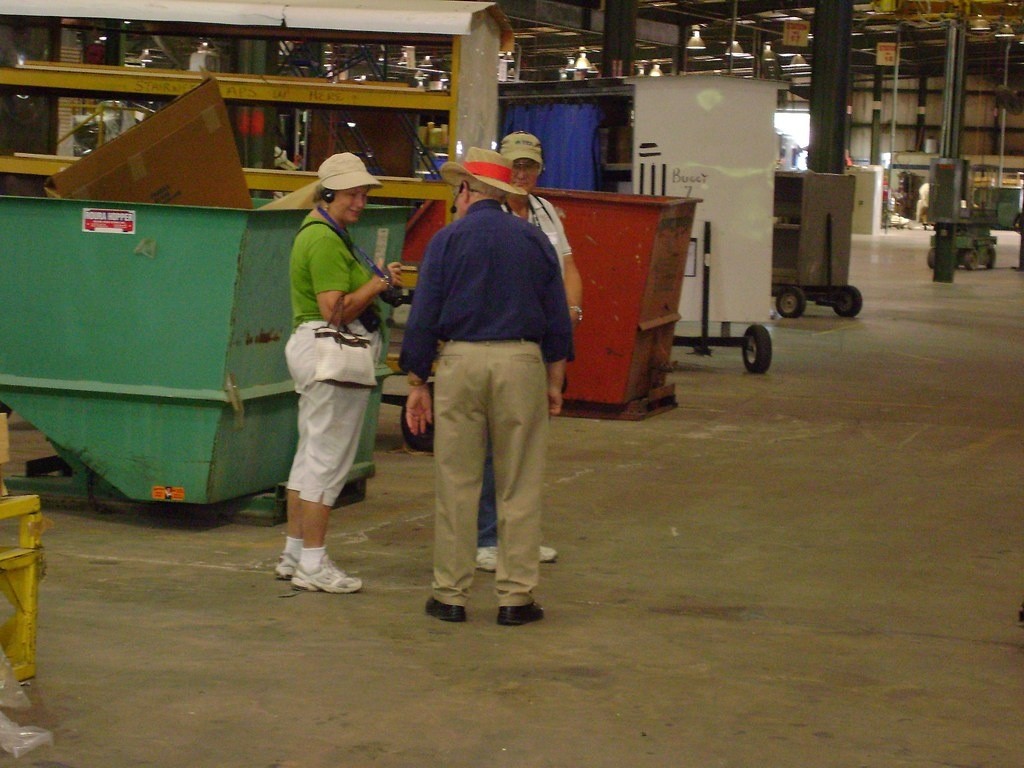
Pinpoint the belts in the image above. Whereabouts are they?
[444,337,539,343]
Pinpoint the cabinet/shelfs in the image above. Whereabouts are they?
[771,168,856,287]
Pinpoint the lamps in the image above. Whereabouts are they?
[970,11,1016,37]
[398,52,449,82]
[503,25,810,77]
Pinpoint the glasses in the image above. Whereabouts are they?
[512,160,536,170]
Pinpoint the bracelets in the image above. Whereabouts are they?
[569,305,583,324]
[407,380,423,386]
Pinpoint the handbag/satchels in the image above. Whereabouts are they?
[314,294,378,390]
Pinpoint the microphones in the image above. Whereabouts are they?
[450,184,463,214]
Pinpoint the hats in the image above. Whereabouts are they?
[440,147,528,196]
[317,153,384,190]
[499,131,543,165]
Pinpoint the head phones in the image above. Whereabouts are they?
[321,188,334,202]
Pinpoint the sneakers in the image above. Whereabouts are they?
[274,552,336,579]
[290,554,362,594]
[473,546,499,571]
[538,544,557,562]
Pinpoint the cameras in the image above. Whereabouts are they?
[380,286,415,307]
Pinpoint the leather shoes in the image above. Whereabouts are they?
[426,598,465,621]
[497,601,544,625]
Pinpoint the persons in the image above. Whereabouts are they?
[396,146,574,626]
[272,153,409,591]
[475,120,585,563]
